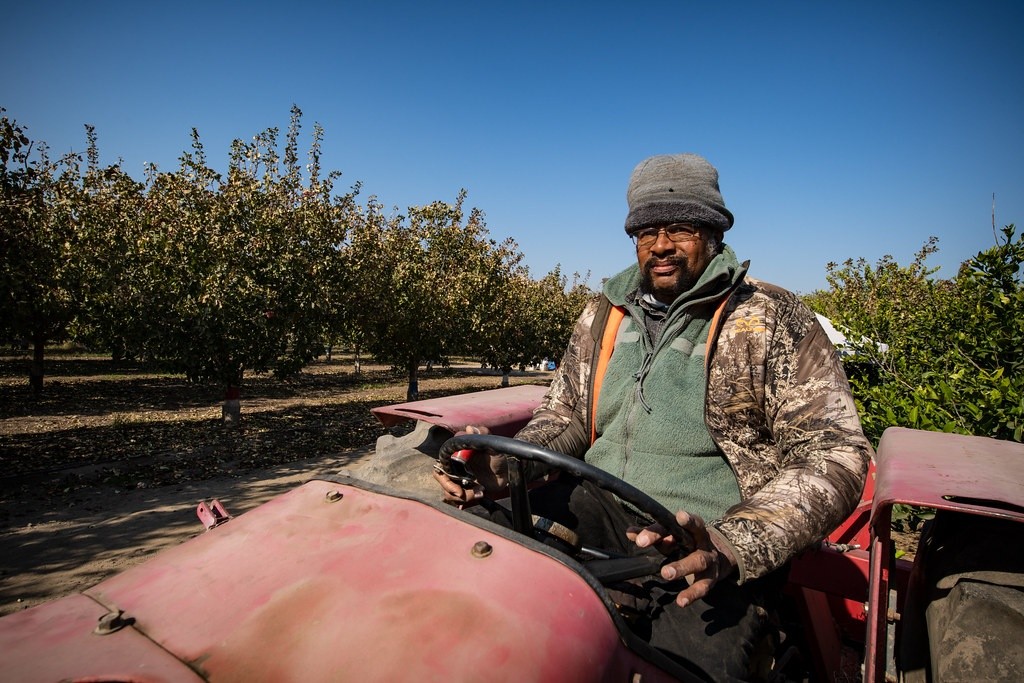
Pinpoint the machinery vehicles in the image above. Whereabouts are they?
[2,380,1024,683]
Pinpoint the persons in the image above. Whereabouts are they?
[434,154,872,683]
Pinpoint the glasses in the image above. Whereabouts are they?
[629,221,706,247]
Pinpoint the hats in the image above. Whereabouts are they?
[624,153,735,238]
